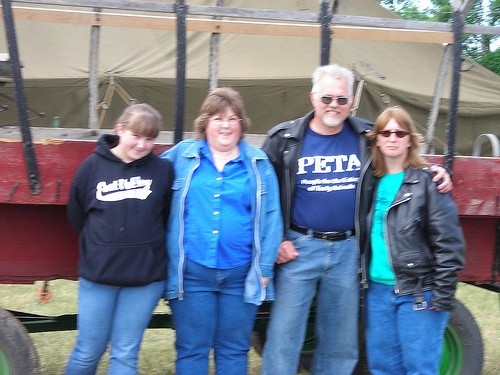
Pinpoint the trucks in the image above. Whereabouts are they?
[0,0,499,374]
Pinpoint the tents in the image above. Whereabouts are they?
[1,1,500,159]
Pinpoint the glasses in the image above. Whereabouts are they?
[208,119,242,126]
[321,96,347,105]
[378,130,410,137]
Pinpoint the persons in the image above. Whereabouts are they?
[258,64,454,374]
[65,101,174,375]
[355,104,467,375]
[158,86,284,375]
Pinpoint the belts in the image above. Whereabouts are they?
[290,221,355,241]
